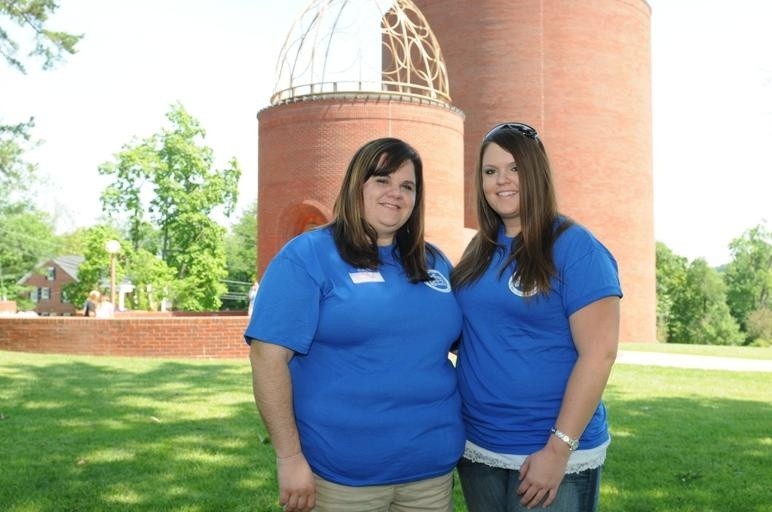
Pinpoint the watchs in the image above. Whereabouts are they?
[549,427,579,450]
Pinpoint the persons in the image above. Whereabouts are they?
[95,295,114,317]
[242,137,462,512]
[454,122,623,512]
[247,279,261,317]
[84,289,99,316]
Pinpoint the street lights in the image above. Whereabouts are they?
[105,240,121,308]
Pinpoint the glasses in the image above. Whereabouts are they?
[483,120,541,146]
